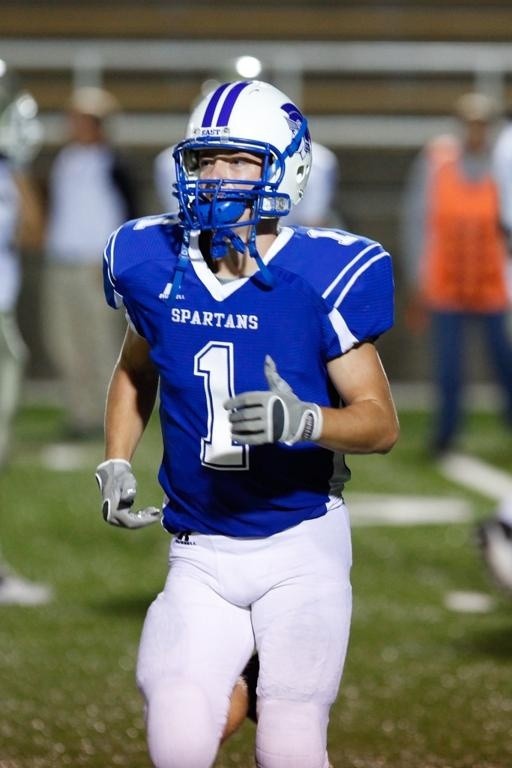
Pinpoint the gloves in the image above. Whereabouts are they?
[92,458,162,530]
[222,355,324,445]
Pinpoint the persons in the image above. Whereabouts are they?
[2,87,130,607]
[94,78,400,767]
[150,123,336,229]
[403,89,512,457]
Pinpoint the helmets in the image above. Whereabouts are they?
[179,80,313,221]
